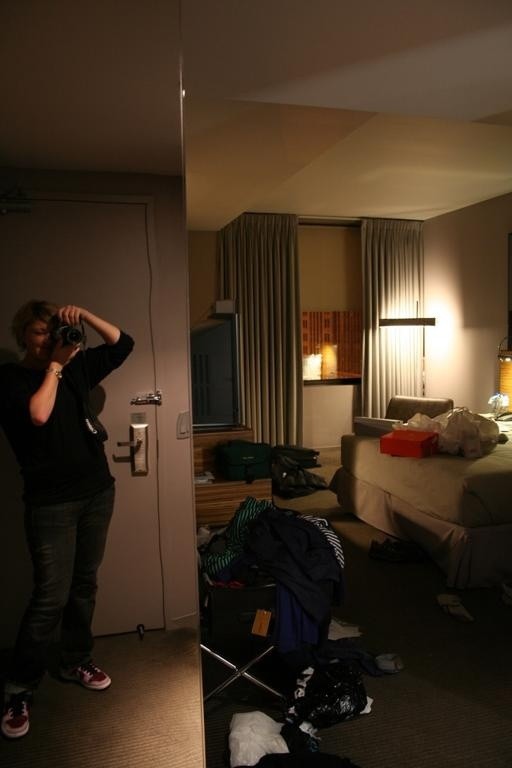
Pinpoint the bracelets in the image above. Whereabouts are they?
[45,367,63,380]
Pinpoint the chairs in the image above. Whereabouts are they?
[352,397,455,439]
[202,583,292,705]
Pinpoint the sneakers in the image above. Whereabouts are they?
[69,664,111,690]
[1,700,30,738]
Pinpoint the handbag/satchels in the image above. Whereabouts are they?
[214,458,274,481]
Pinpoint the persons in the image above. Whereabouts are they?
[1,300,136,738]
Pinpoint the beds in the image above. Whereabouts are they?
[330,396,512,580]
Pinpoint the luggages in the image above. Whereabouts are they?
[275,446,321,468]
[216,440,272,461]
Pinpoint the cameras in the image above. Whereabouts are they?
[48,314,84,345]
